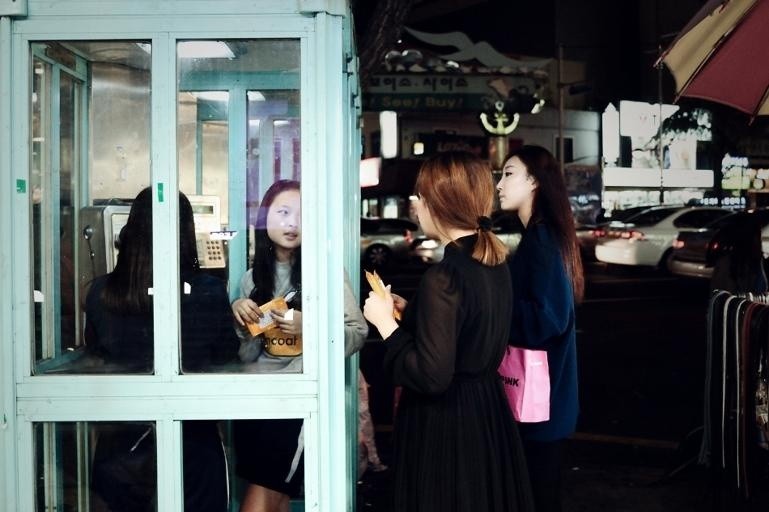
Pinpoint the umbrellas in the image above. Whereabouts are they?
[650,0,769,126]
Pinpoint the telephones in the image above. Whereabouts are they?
[119,224,128,240]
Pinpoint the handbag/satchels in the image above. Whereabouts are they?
[499,345,552,425]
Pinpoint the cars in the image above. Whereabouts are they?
[594,202,768,280]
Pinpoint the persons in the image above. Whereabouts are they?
[83,184,240,512]
[362,150,536,511]
[229,179,369,512]
[495,142,586,511]
[709,214,769,331]
